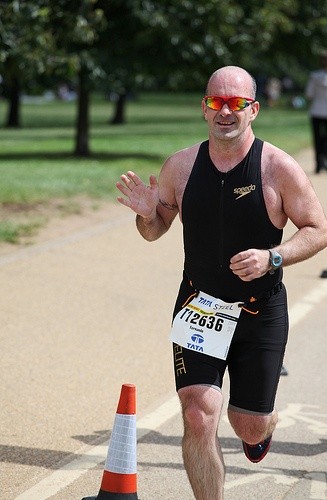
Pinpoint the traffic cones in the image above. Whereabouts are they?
[81,383,142,500]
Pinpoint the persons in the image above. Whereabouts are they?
[254,70,305,109]
[116,66,327,500]
[305,55,327,175]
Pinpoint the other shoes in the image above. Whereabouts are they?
[242,436,272,463]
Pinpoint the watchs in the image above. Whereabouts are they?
[267,249,282,275]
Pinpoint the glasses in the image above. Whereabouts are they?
[203,95,254,112]
